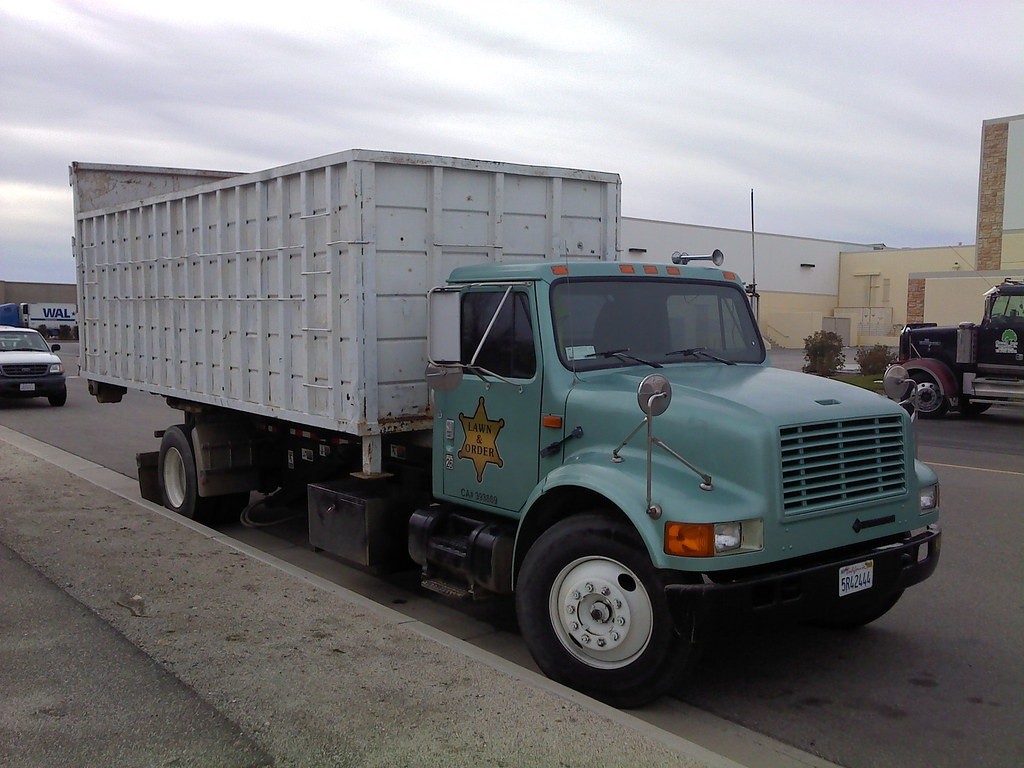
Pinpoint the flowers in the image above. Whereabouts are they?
[801,329,846,374]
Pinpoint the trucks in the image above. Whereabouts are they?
[893,278,1024,420]
[66,148,946,711]
[0,301,77,340]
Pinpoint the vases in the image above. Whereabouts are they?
[816,356,831,373]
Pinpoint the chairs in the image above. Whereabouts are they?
[594,293,671,357]
[16,340,30,348]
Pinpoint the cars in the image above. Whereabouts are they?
[0,324,68,407]
